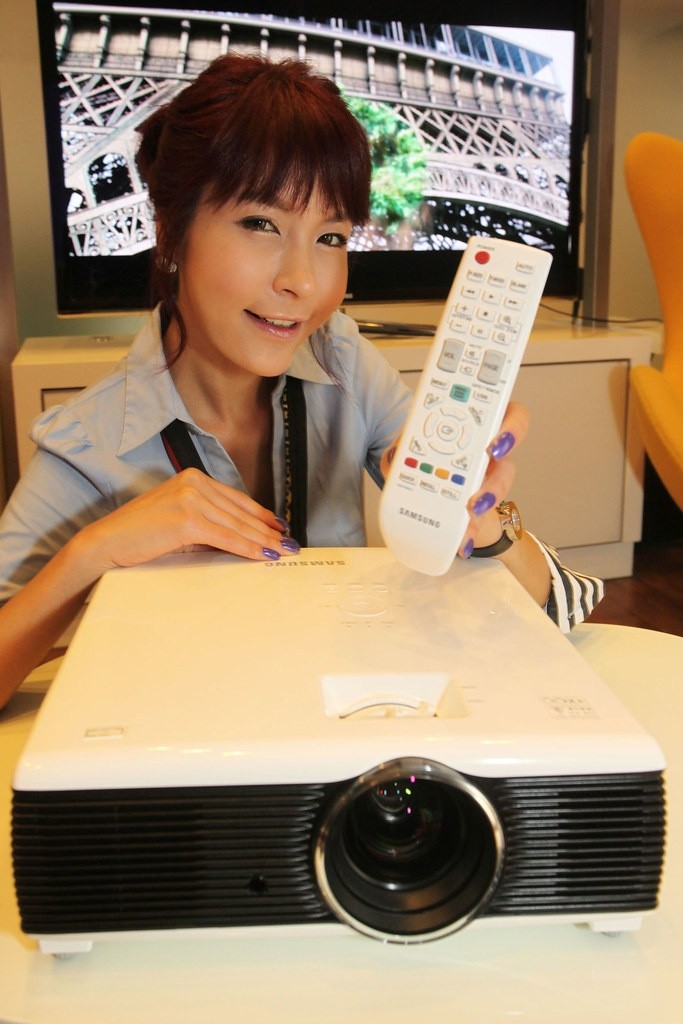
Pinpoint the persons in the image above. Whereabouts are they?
[0,56,604,710]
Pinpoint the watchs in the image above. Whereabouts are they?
[471,501,522,558]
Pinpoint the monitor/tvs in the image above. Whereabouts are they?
[32,0,589,337]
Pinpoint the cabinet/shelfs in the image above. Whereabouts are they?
[11,327,652,581]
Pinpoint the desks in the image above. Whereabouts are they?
[0,604,683,1024]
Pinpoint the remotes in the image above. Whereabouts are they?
[378,236,554,576]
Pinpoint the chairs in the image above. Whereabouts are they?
[627,131,683,510]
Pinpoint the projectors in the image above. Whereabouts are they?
[7,547,670,953]
